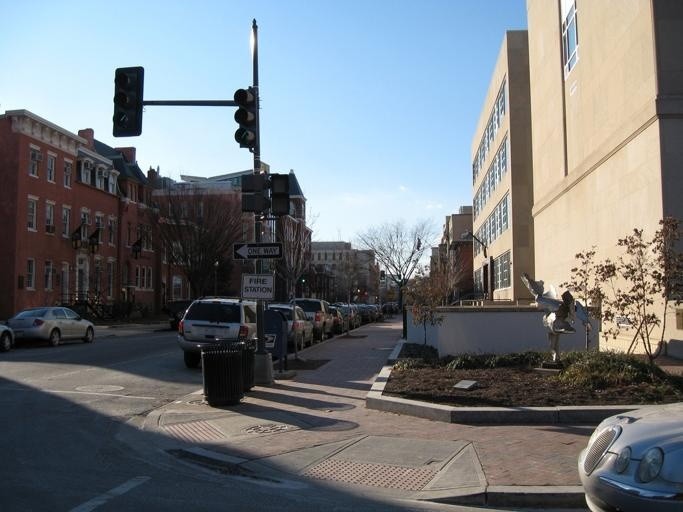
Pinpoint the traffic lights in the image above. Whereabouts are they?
[234,87,259,147]
[113,72,131,126]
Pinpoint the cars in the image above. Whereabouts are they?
[577,401,682,512]
[1,307,95,352]
[177,295,399,367]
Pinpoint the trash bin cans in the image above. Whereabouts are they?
[196,337,259,407]
